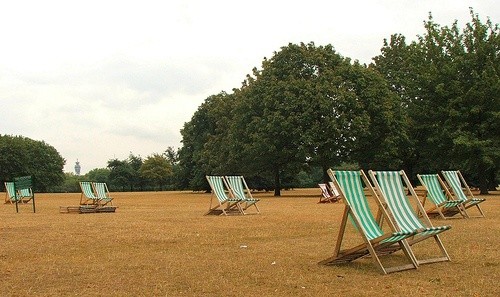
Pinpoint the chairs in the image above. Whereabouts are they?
[79,182,114,206]
[318,169,486,275]
[203,175,261,216]
[4,181,33,208]
